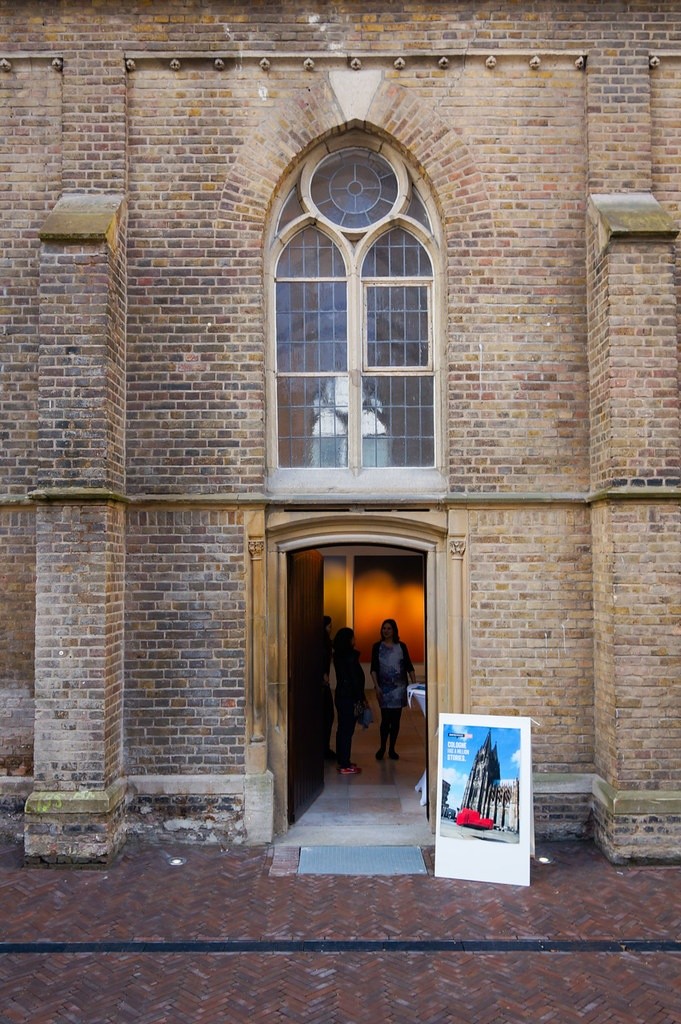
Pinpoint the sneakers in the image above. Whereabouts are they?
[335,763,362,775]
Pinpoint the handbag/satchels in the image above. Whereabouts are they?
[353,700,374,729]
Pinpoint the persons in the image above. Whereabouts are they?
[370,618,417,760]
[323,615,338,762]
[331,628,370,774]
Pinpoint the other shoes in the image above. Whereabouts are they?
[389,747,399,760]
[325,749,337,762]
[375,747,386,761]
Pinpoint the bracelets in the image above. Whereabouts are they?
[374,682,379,689]
